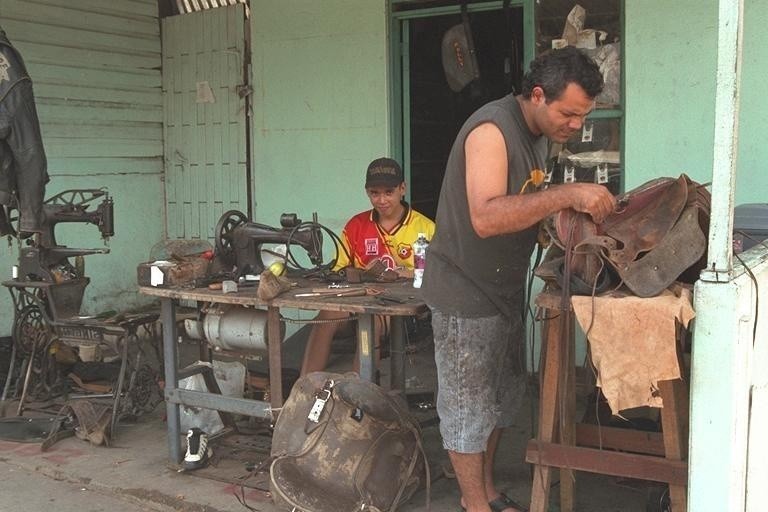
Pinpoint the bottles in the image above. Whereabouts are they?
[412,233,427,289]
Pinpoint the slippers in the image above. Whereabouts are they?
[326,259,406,283]
[461,490,528,512]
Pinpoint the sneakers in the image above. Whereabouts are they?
[184,427,208,464]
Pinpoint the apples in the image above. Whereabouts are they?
[270,263,287,277]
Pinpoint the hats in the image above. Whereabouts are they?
[364,157,403,187]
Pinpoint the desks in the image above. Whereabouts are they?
[144,271,433,462]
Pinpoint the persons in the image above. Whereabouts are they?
[299,158,435,379]
[420,45,618,512]
